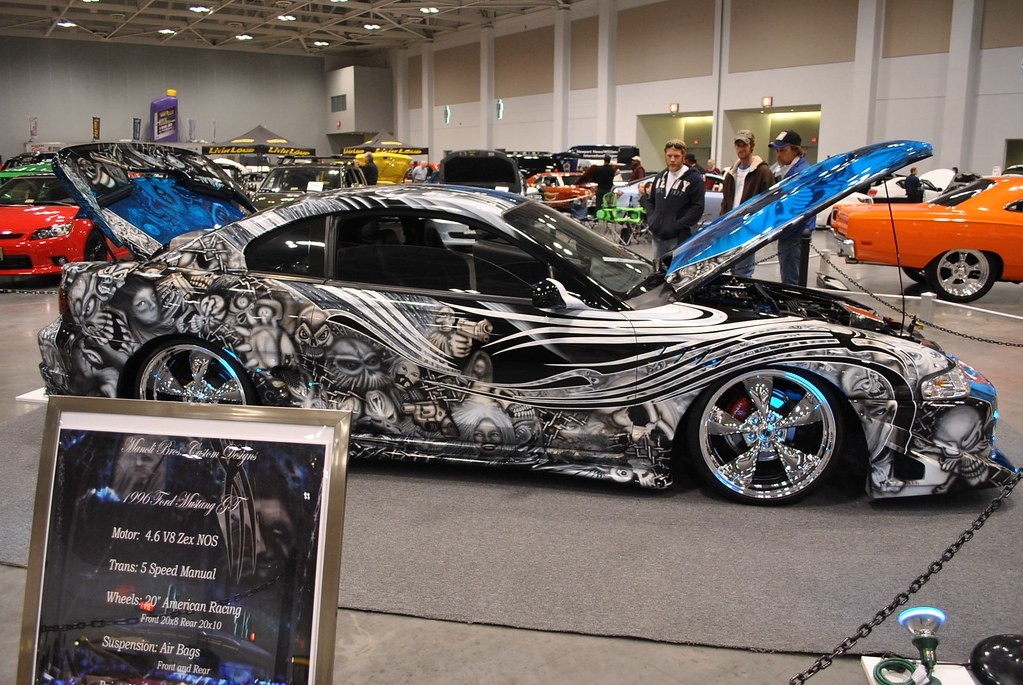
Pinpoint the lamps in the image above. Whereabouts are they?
[898,604,946,674]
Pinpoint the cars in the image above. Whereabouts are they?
[0,149,137,287]
[609,171,725,226]
[212,145,655,251]
[814,166,1023,304]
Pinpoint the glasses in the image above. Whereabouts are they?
[665,143,687,150]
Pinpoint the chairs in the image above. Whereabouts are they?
[367,227,411,287]
[413,219,451,279]
[569,192,652,247]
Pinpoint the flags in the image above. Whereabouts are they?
[30,117,38,137]
[189,119,195,140]
[93,117,100,140]
[133,118,141,140]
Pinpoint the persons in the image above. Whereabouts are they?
[359,152,378,185]
[629,155,645,184]
[705,159,721,190]
[402,160,433,183]
[768,129,816,287]
[619,181,653,246]
[646,138,705,260]
[952,167,958,174]
[684,153,706,174]
[904,167,921,201]
[592,151,616,217]
[718,129,776,278]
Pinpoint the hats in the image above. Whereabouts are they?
[685,153,695,161]
[733,129,756,145]
[768,130,801,148]
[631,155,641,162]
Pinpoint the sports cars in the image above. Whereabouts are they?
[36,138,1023,507]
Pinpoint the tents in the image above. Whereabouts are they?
[202,125,317,176]
[339,128,429,163]
